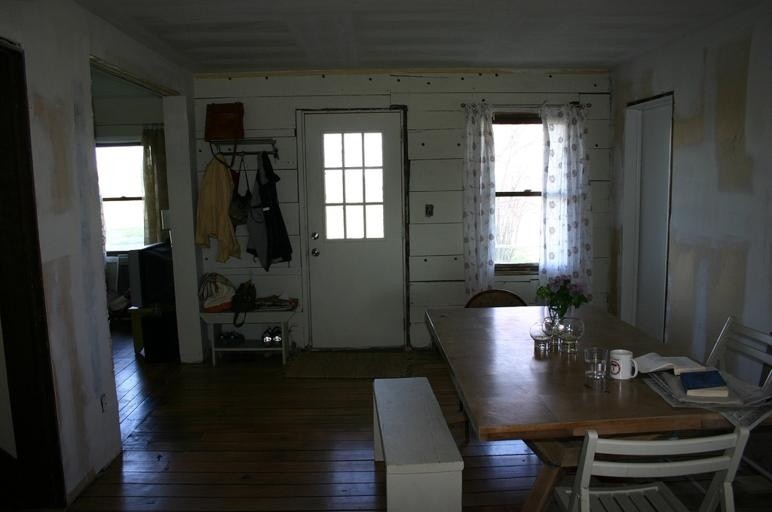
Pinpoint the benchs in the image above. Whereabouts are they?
[372,378,465,511]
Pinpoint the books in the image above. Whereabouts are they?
[631,351,706,376]
[680,369,728,397]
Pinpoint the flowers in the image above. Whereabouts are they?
[535,273,593,330]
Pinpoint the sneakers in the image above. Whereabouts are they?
[215,331,245,348]
[262,325,282,348]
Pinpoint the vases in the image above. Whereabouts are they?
[541,317,558,336]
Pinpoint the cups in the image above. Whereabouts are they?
[555,317,586,342]
[609,349,640,380]
[529,321,555,341]
[584,345,608,380]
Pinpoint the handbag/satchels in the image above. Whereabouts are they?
[200,272,234,313]
[228,189,253,226]
[203,101,245,144]
[231,280,255,314]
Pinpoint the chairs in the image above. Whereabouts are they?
[553,424,750,511]
[464,289,528,308]
[663,315,772,444]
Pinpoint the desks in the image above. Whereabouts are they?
[199,299,295,368]
[129,306,159,353]
[424,303,771,511]
